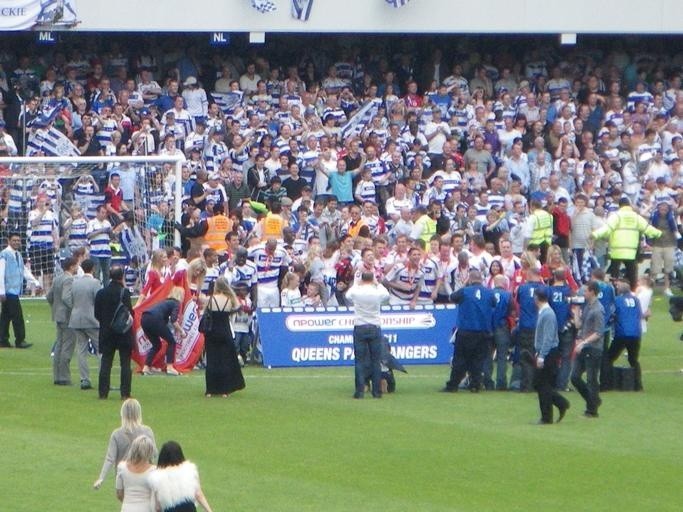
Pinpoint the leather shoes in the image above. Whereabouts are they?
[556,402,570,422]
[167,369,183,376]
[143,369,153,376]
[471,386,479,393]
[16,340,32,348]
[81,385,93,389]
[354,392,364,399]
[372,393,381,398]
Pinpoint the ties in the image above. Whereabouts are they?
[15,252,20,265]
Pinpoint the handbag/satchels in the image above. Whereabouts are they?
[198,294,213,333]
[109,288,134,335]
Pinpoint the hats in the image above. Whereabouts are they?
[519,80,529,90]
[207,172,220,180]
[584,161,595,168]
[196,117,210,128]
[182,76,197,86]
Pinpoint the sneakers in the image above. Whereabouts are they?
[673,231,682,240]
[663,287,675,297]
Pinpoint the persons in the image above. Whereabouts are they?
[1,31,681,296]
[2,232,682,422]
[149,440,213,512]
[116,435,158,512]
[94,398,159,490]
